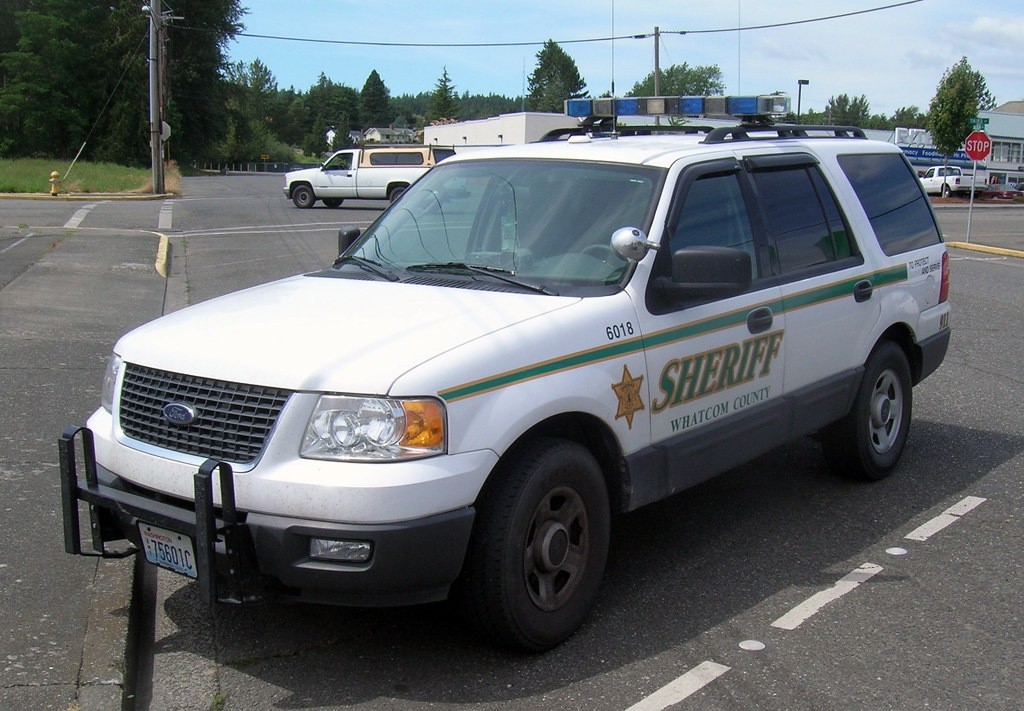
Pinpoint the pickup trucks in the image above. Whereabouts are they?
[282,144,470,210]
[918,166,989,198]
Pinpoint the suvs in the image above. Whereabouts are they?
[50,96,953,663]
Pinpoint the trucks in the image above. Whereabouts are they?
[422,113,596,157]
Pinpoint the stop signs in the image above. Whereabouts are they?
[964,130,991,162]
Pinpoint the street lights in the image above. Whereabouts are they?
[795,79,810,126]
